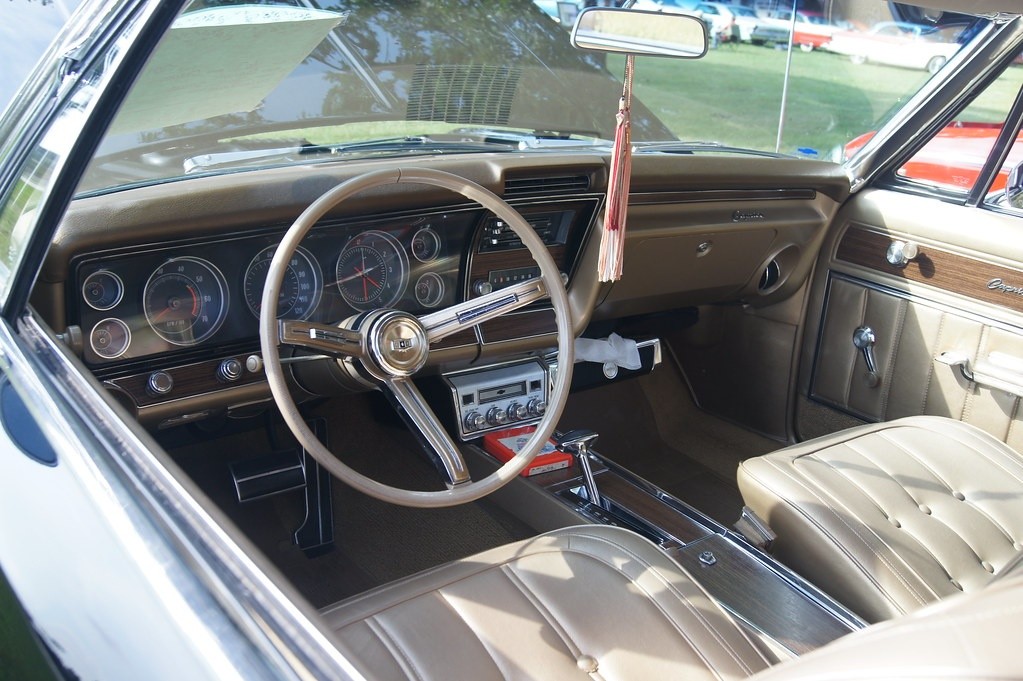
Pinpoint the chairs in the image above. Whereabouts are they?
[736,415,1023,625]
[317,525,1023,681]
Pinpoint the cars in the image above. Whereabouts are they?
[534,1,864,53]
[828,21,964,75]
[821,119,1023,209]
[0,0,1023,681]
